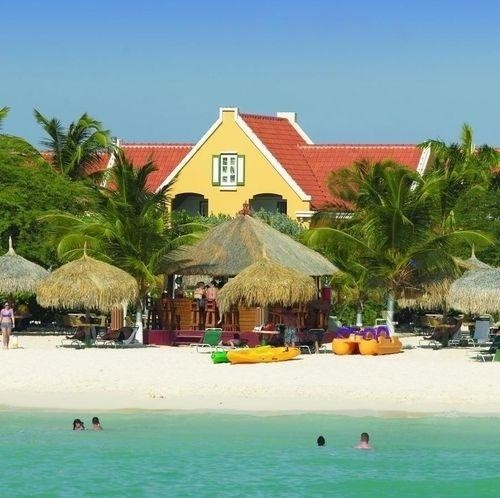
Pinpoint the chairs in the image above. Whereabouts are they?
[294,327,325,355]
[61,325,139,350]
[473,336,500,363]
[189,327,223,356]
[418,320,462,351]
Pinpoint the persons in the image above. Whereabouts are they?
[0,302,16,349]
[317,435,325,446]
[356,432,373,450]
[73,420,84,430]
[92,417,103,430]
[193,280,218,303]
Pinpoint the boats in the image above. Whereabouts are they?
[332,334,402,355]
[211,345,301,364]
